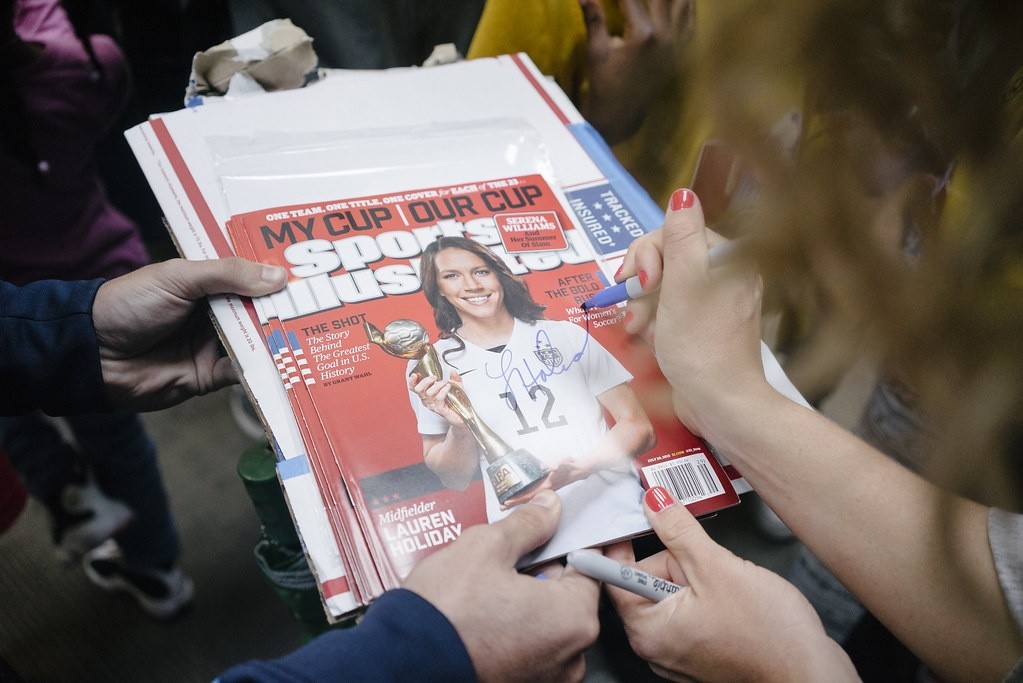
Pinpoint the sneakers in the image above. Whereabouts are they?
[83,538,193,614]
[49,496,101,552]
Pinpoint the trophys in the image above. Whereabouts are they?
[363,318,550,505]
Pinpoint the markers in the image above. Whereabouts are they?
[581,230,750,311]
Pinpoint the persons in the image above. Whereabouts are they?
[0,1,603,683]
[405,236,657,569]
[466,0,1023,683]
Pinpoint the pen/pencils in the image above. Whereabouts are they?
[564,548,684,604]
